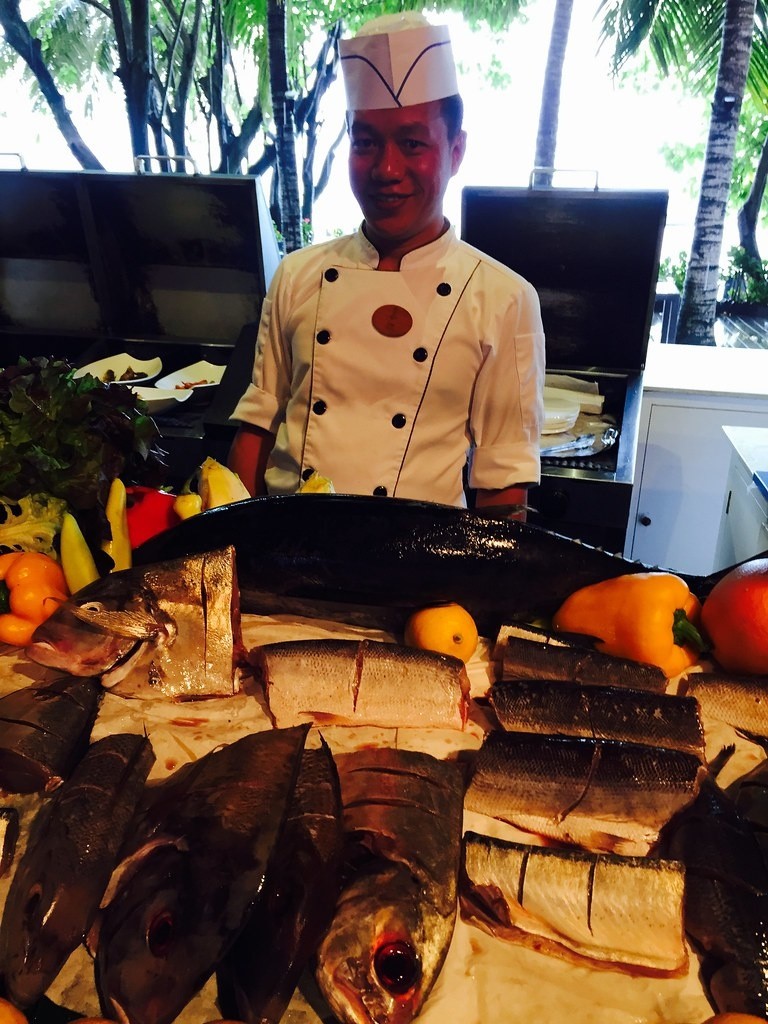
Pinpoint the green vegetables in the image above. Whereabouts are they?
[0,354,174,614]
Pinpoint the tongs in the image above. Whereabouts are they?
[541,432,597,458]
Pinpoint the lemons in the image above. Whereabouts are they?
[403,601,478,663]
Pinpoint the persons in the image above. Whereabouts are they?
[228,8,546,523]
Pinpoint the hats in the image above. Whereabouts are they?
[338,23,458,109]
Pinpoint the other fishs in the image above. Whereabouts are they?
[0,493,767,1024]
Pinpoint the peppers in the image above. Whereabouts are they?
[0,479,206,646]
[551,571,705,675]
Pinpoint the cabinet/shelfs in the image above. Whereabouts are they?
[623,298,768,576]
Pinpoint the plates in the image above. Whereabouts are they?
[127,386,194,414]
[542,395,579,433]
[155,360,227,393]
[71,353,163,389]
